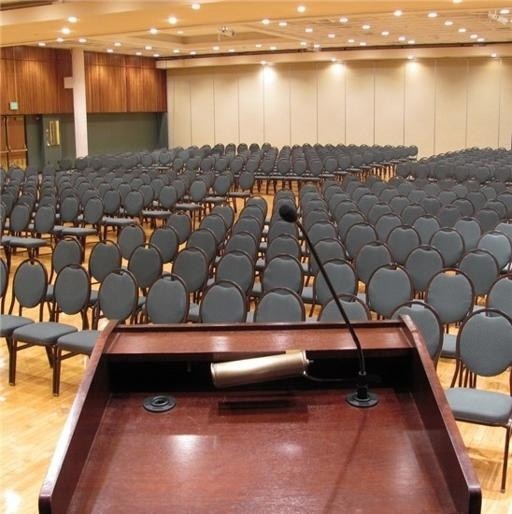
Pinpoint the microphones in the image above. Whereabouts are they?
[279,205,379,408]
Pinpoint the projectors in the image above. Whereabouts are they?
[217,30,236,38]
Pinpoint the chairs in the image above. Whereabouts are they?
[0,146,188,273]
[188,143,417,197]
[418,149,512,195]
